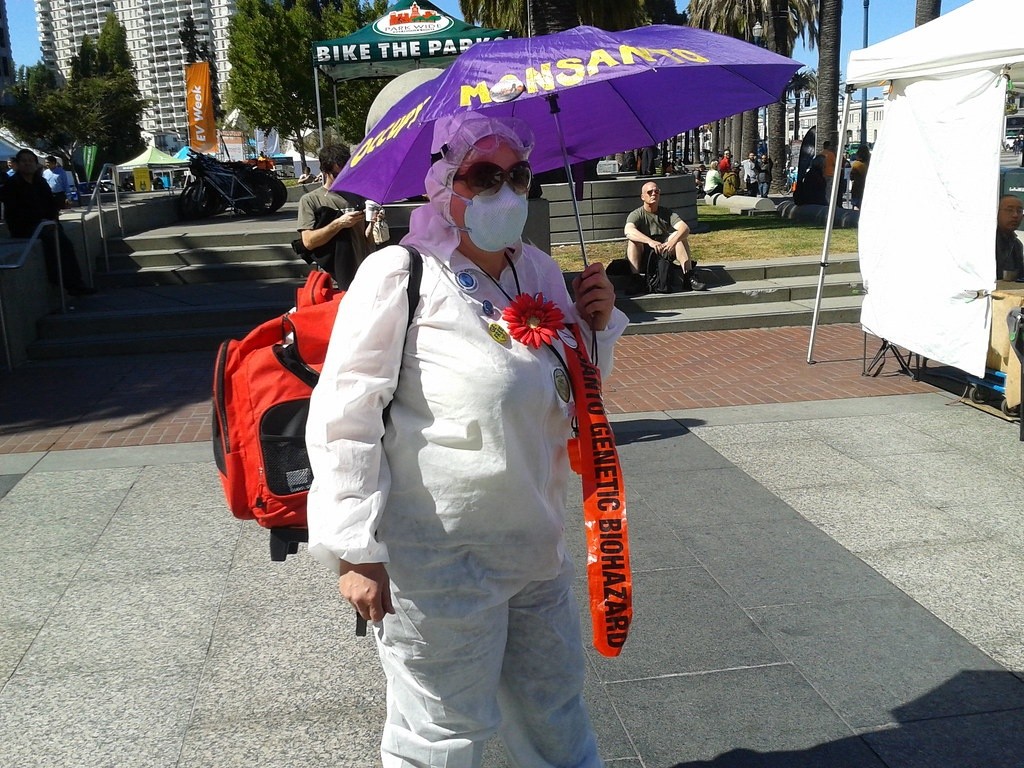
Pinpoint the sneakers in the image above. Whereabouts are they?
[625,273,645,296]
[680,270,707,290]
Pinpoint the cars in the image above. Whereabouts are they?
[65,179,117,203]
[846,142,861,156]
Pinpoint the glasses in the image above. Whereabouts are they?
[646,189,661,195]
[327,169,338,178]
[999,206,1024,214]
[454,161,534,197]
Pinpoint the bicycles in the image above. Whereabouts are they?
[777,169,796,196]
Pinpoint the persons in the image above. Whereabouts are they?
[258,151,267,161]
[623,181,705,290]
[41,156,73,207]
[122,172,192,191]
[996,195,1024,280]
[303,106,631,768]
[794,140,870,207]
[703,148,774,197]
[297,167,315,183]
[298,144,383,283]
[0,149,99,296]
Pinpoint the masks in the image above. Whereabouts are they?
[450,182,528,254]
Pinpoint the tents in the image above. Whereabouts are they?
[806,0,1024,364]
[116,142,192,190]
[311,0,519,184]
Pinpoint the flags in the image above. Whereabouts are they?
[184,62,218,155]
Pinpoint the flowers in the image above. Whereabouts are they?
[502,292,565,350]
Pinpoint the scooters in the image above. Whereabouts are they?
[174,147,289,222]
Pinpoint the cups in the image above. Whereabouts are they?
[1002,269,1018,281]
[365,200,378,222]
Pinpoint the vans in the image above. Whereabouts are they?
[1005,135,1019,151]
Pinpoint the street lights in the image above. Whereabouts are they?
[752,20,764,46]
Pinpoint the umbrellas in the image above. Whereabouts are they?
[328,23,804,316]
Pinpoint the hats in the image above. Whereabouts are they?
[731,160,740,168]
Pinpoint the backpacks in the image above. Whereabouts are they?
[646,249,673,293]
[212,243,422,562]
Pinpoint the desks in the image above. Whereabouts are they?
[967,280,1024,417]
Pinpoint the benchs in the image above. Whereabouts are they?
[704,193,776,217]
[775,201,861,230]
